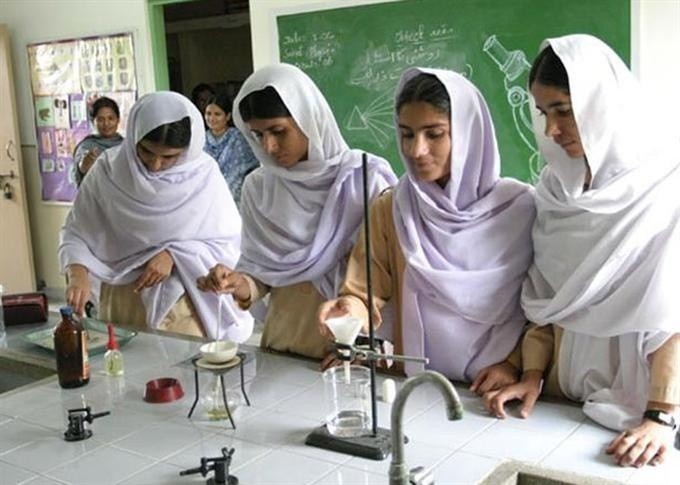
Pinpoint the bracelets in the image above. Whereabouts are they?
[643,408,677,432]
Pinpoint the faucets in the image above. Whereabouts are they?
[387,368,466,485]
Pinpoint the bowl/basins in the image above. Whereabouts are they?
[199,339,239,364]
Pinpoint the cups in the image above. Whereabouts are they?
[322,365,375,438]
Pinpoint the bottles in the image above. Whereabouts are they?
[53,307,90,389]
[200,374,243,422]
[103,324,124,377]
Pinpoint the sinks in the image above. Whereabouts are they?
[478,456,616,485]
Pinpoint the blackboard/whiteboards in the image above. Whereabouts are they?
[274,0,631,187]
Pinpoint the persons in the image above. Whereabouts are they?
[71,95,128,191]
[193,60,402,369]
[201,93,260,206]
[316,63,540,399]
[191,83,215,116]
[480,32,680,471]
[55,87,255,345]
